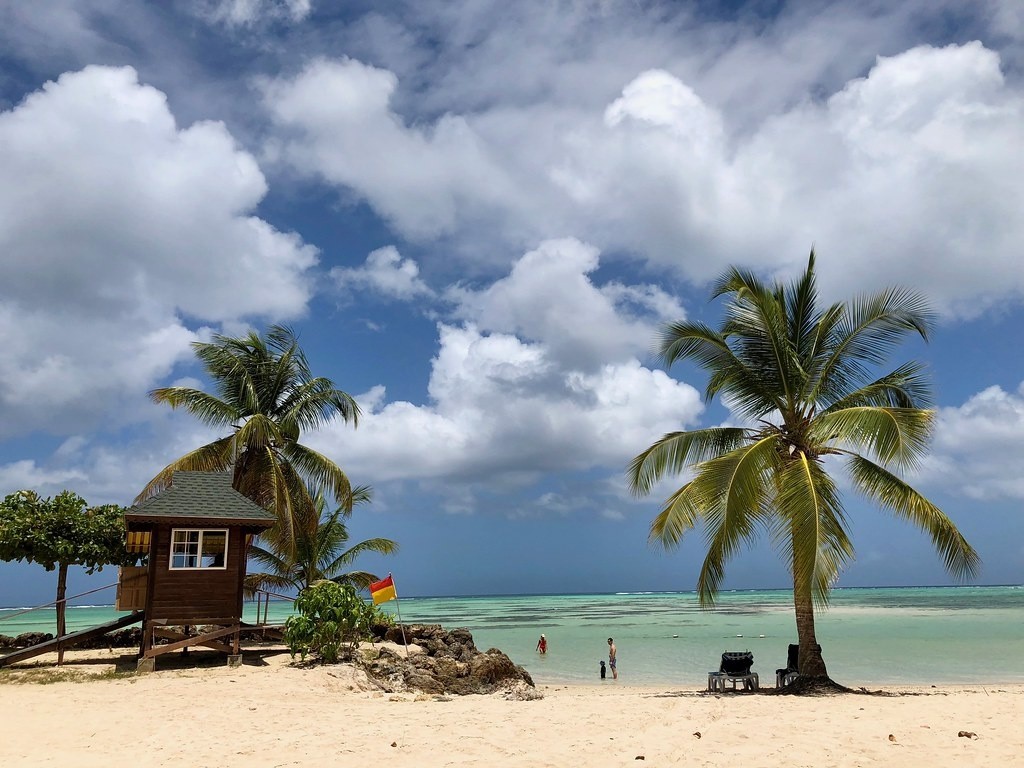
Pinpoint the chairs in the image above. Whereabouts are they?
[707,649,759,692]
[776,643,822,692]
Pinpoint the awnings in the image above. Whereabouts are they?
[125,531,254,556]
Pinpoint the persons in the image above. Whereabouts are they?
[536,634,548,654]
[600,661,606,678]
[607,638,617,679]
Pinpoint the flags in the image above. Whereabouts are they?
[369,575,398,607]
